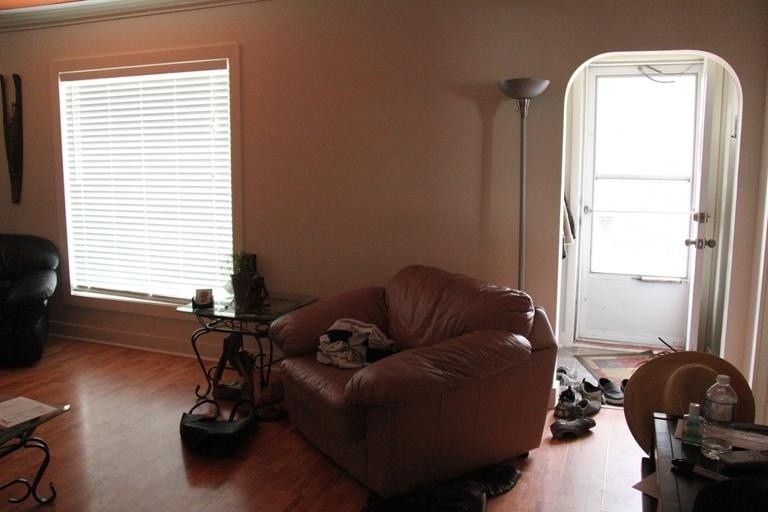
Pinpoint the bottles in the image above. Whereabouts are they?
[682,403,704,446]
[702,374,737,460]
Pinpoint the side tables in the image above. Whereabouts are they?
[176,292,319,413]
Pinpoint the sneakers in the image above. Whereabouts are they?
[550,366,630,440]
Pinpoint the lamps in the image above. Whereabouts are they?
[500,78,550,294]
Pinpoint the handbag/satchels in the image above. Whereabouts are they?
[180,399,258,460]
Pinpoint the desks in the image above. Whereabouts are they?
[1,395,71,510]
[641,411,768,512]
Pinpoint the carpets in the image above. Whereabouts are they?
[573,350,659,390]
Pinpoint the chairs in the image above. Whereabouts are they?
[0,235,62,369]
[269,263,558,499]
